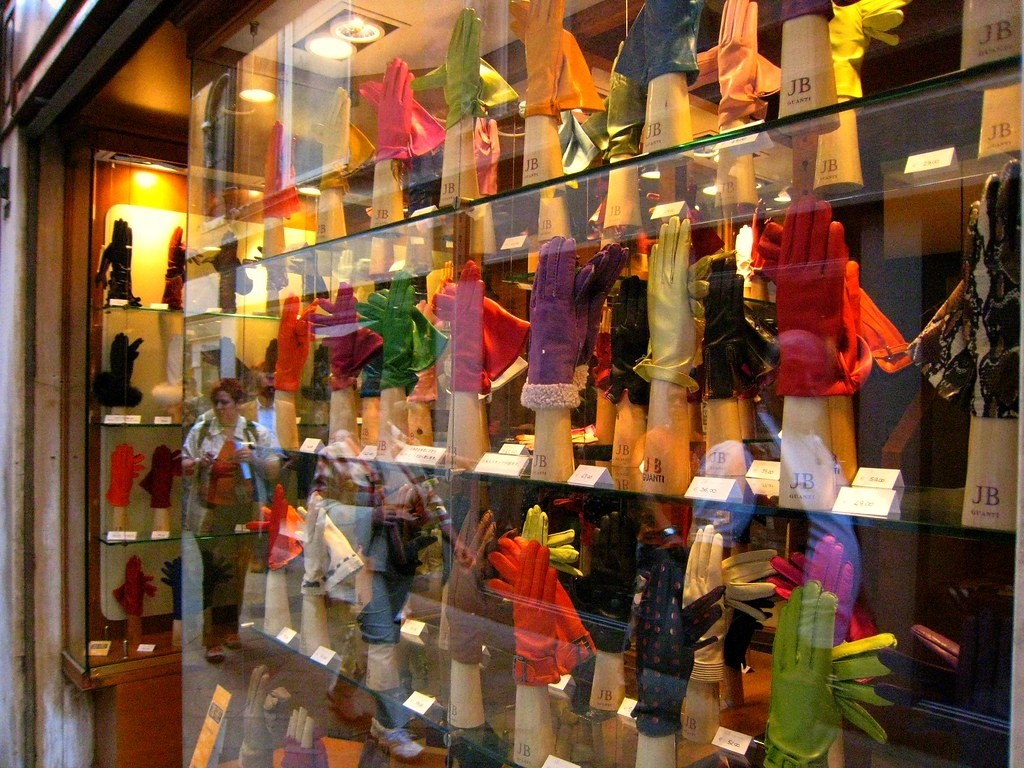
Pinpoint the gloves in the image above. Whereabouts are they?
[717,0,768,128]
[829,1,872,98]
[109,505,1018,768]
[961,157,1018,418]
[778,0,860,32]
[903,278,978,410]
[862,1,911,46]
[775,195,859,397]
[687,45,781,97]
[757,223,916,374]
[60,0,775,441]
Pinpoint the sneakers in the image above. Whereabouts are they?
[369,717,426,763]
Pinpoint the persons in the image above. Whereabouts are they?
[94,0,1024,767]
[180,364,300,661]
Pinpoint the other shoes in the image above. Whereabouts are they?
[224,635,242,651]
[206,646,224,662]
[324,701,371,742]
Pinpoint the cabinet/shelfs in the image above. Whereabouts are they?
[62,50,1024,768]
